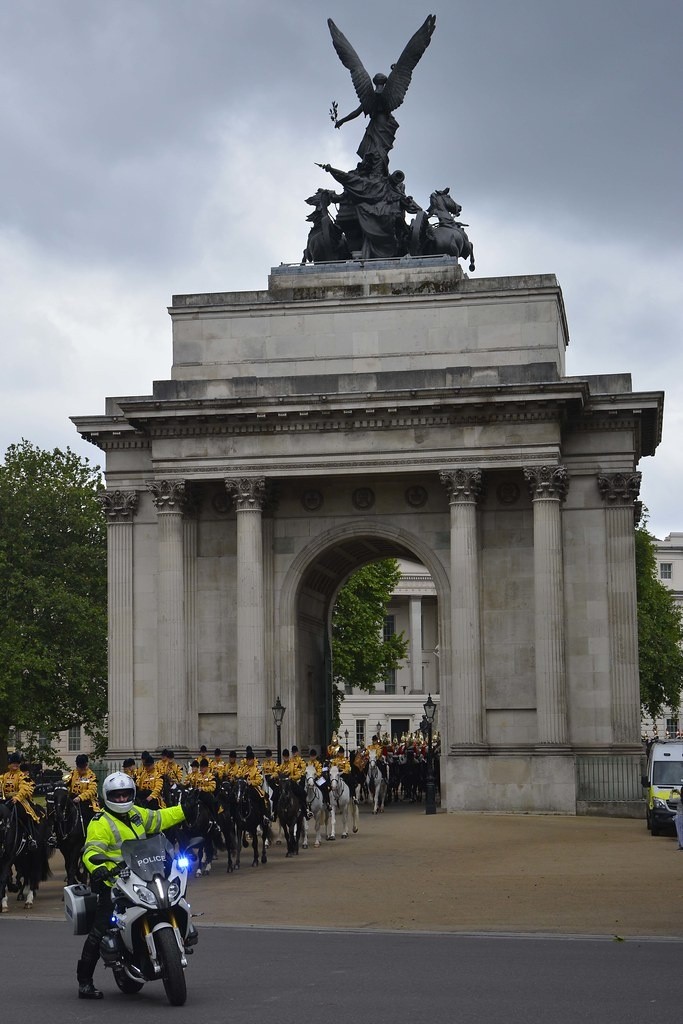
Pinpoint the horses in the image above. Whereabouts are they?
[0,735,443,914]
[296,205,478,273]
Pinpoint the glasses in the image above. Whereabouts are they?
[111,793,129,798]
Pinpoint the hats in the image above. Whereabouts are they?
[8,753,22,764]
[189,745,318,769]
[328,730,440,753]
[75,754,89,768]
[121,748,175,767]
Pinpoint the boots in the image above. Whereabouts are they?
[76,959,104,1000]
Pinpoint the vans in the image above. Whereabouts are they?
[641,739,683,838]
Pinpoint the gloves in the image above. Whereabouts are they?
[184,787,200,819]
[91,866,116,894]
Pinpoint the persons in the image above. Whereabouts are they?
[355,731,442,791]
[122,729,359,831]
[47,754,101,845]
[77,773,199,1000]
[334,73,400,159]
[0,753,57,849]
[669,787,683,851]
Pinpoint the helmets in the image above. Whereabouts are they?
[102,772,137,813]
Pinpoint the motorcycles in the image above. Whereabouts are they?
[63,836,207,1007]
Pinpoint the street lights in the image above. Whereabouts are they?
[420,693,437,814]
[400,685,409,695]
[376,720,382,741]
[271,696,287,767]
[344,728,349,758]
